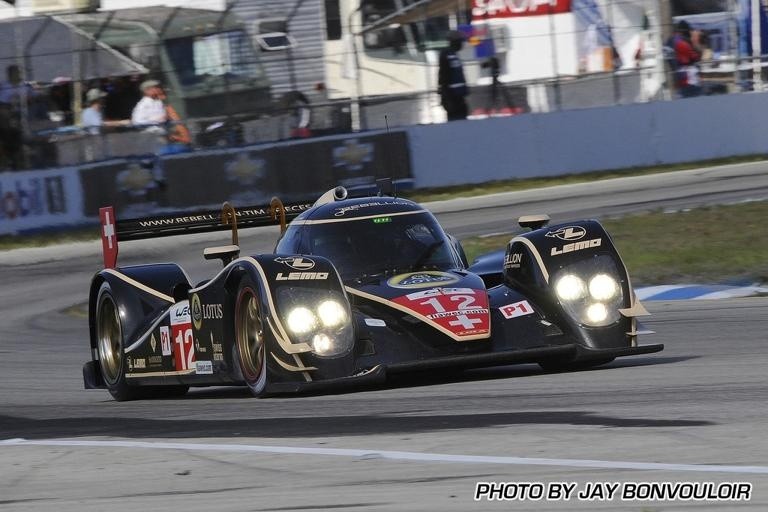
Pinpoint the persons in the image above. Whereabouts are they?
[130,78,169,131]
[667,23,701,97]
[0,64,36,103]
[77,88,108,135]
[438,30,475,122]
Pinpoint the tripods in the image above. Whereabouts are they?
[486,69,517,114]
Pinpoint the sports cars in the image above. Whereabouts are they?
[82,177,663,401]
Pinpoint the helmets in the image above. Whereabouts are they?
[447,30,466,41]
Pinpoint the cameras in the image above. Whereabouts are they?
[482,57,498,68]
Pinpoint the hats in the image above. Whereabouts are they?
[86,89,109,100]
[140,79,160,92]
[677,20,689,32]
